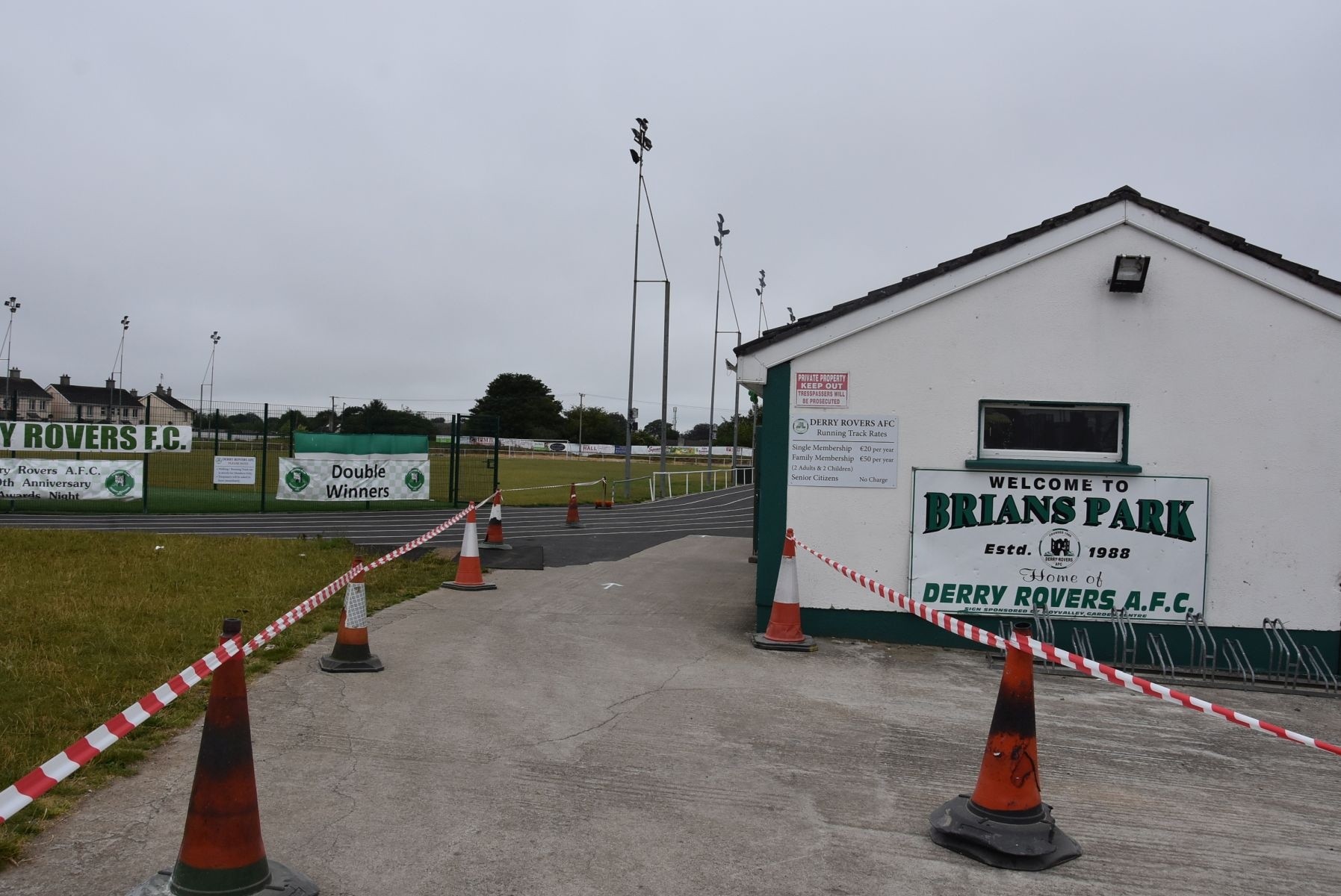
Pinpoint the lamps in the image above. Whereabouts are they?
[1106,251,1152,293]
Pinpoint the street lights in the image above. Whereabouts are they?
[209,331,221,433]
[5,295,23,424]
[121,315,132,424]
[621,113,657,506]
[703,214,729,486]
[751,268,767,337]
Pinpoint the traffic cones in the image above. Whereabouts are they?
[559,483,589,531]
[750,527,818,653]
[142,614,320,896]
[313,554,387,672]
[476,487,513,551]
[439,500,501,591]
[928,614,1086,871]
